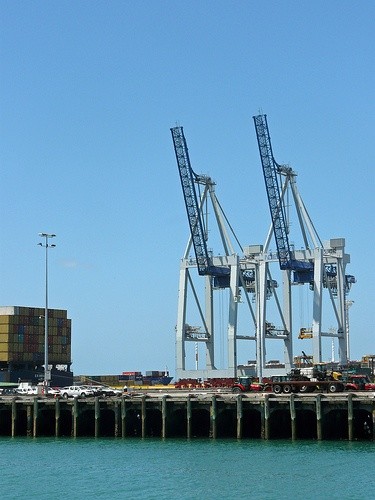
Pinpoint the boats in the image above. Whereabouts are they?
[79,371,173,385]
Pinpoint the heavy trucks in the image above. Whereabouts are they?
[232,372,371,393]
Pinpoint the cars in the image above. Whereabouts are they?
[17,382,121,399]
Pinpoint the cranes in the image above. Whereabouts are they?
[171,114,355,381]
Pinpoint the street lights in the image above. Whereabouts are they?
[36,232,56,397]
